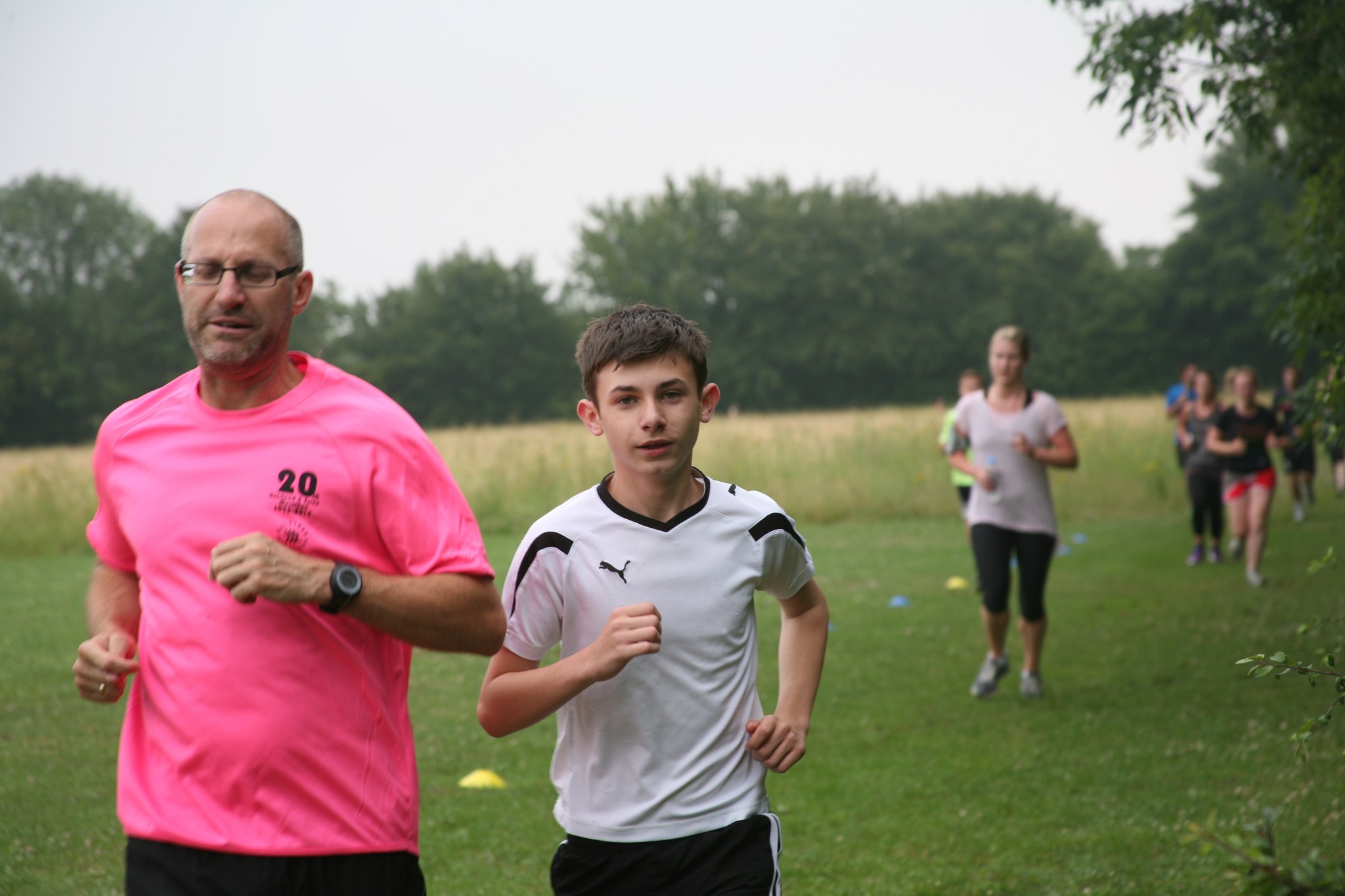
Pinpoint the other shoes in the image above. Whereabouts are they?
[1186,543,1204,568]
[1018,669,1044,700]
[1244,567,1265,587]
[1230,537,1244,558]
[969,655,1010,701]
[1208,543,1221,562]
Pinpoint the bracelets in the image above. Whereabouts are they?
[1028,446,1037,459]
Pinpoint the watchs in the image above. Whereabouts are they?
[318,560,362,614]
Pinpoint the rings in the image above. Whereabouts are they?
[99,683,107,698]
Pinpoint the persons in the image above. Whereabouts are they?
[479,303,828,896]
[950,326,1105,701]
[73,189,510,896]
[938,358,1345,594]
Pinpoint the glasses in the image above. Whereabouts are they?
[177,262,299,288]
